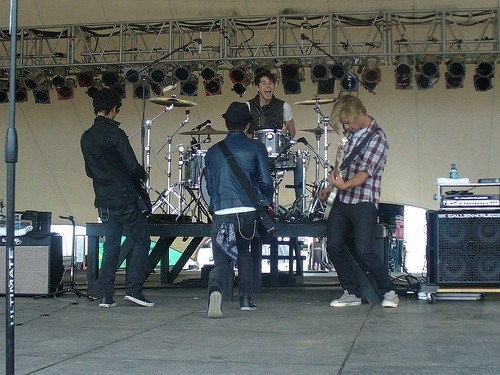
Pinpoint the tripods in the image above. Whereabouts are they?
[32,215,94,302]
[151,102,340,221]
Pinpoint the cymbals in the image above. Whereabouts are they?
[179,127,228,135]
[294,98,337,105]
[149,96,197,107]
[299,127,334,135]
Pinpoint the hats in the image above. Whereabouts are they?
[221,102,251,124]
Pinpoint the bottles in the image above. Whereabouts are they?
[449,164,458,179]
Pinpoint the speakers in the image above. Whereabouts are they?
[426,209,500,287]
[0,235,63,296]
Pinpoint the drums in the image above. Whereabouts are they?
[272,139,297,170]
[199,163,211,210]
[184,148,207,190]
[255,129,291,161]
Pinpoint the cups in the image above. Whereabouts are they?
[14,214,21,230]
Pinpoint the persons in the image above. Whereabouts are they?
[245,71,297,145]
[318,95,400,307]
[204,101,274,318]
[81,89,156,308]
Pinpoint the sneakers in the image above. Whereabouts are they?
[99,297,117,308]
[207,287,224,317]
[329,289,362,306]
[124,291,155,307]
[381,290,400,308]
[239,295,257,310]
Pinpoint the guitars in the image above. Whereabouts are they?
[323,136,349,221]
[127,178,152,215]
